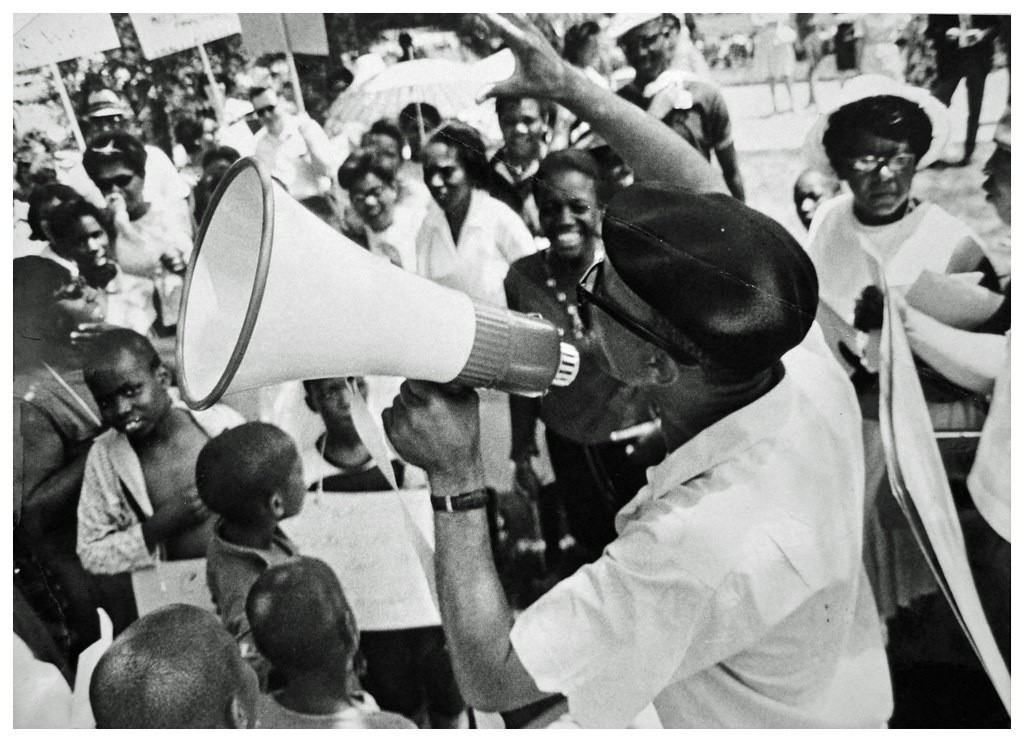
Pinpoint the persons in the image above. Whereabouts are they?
[380,13,895,729]
[14,13,1010,729]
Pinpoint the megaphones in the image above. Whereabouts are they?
[176,154,581,401]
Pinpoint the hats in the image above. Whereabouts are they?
[601,180,821,358]
[802,73,952,171]
[606,13,662,39]
[87,89,124,118]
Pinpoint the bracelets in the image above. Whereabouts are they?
[430,489,489,512]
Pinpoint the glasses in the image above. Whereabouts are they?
[96,174,137,190]
[623,31,664,53]
[574,260,702,367]
[256,104,277,117]
[91,116,122,129]
[838,150,914,172]
